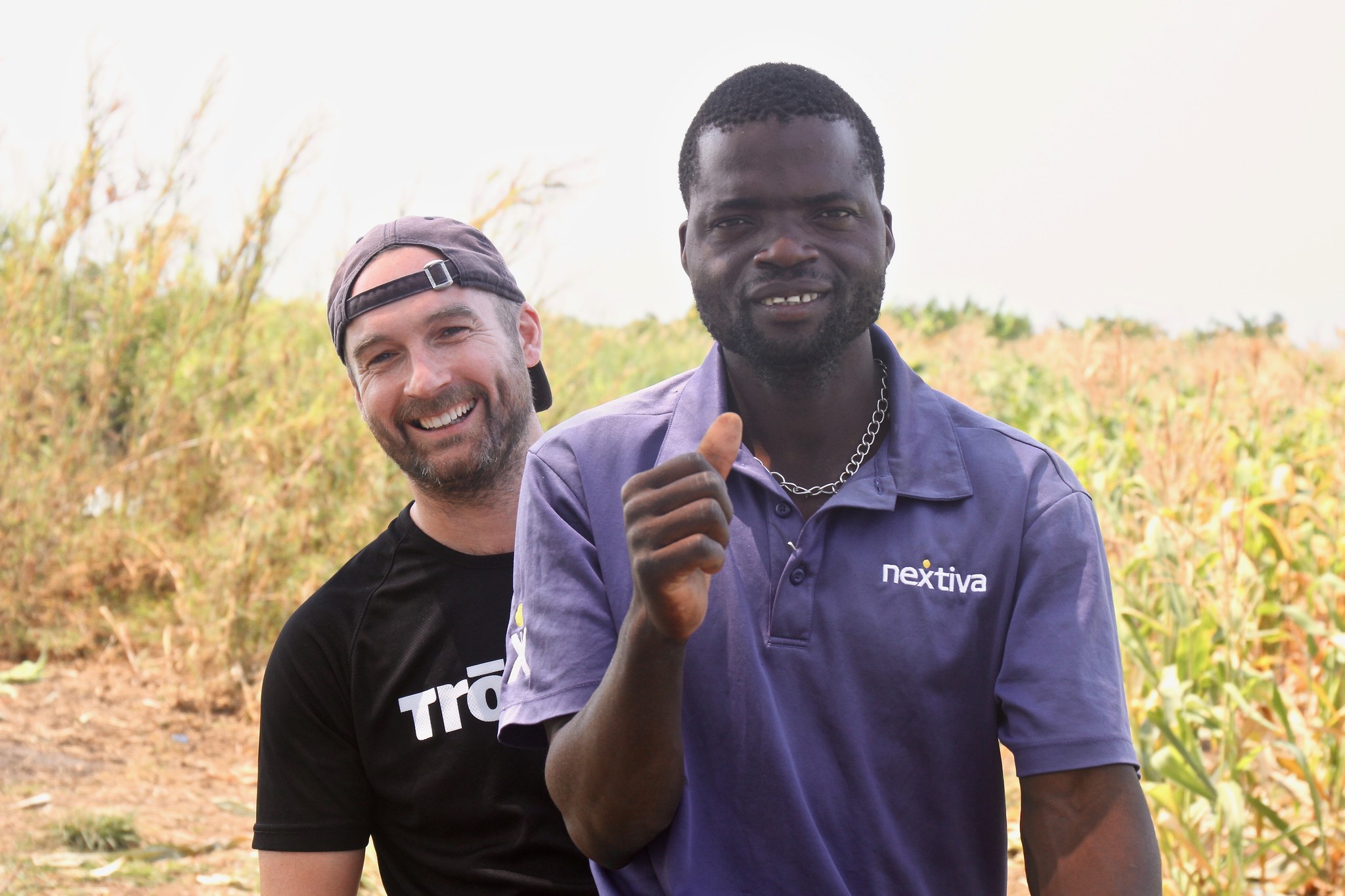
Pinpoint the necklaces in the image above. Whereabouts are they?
[719,356,892,499]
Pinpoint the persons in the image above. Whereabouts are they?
[245,214,600,896]
[491,63,1163,896]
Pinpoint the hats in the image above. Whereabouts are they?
[326,216,554,413]
